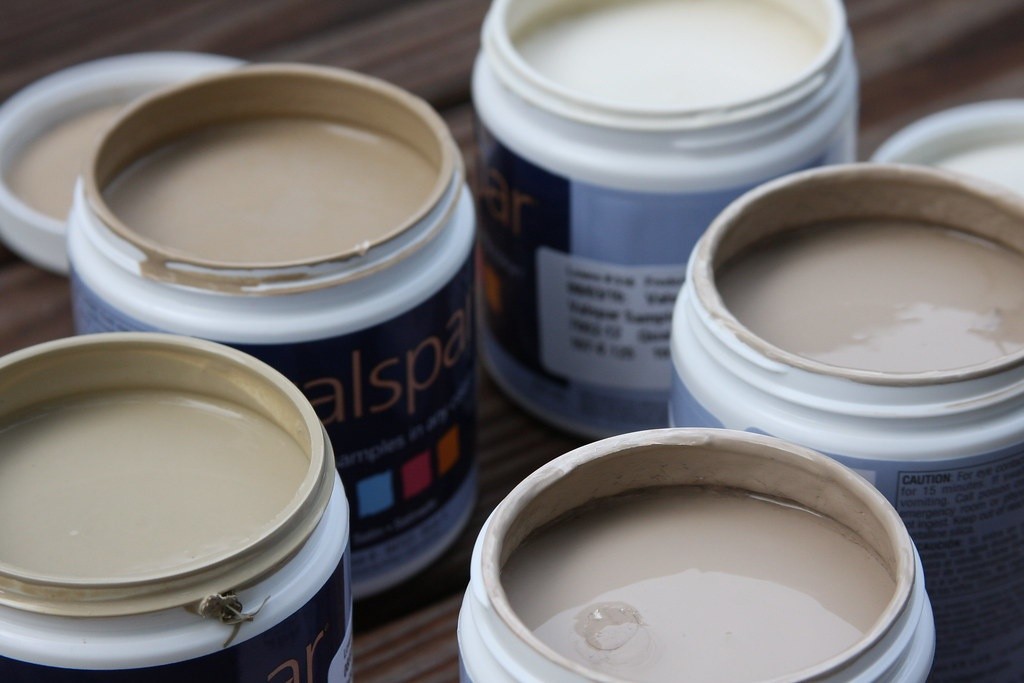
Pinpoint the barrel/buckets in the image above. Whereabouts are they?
[0,0,1024,683]
